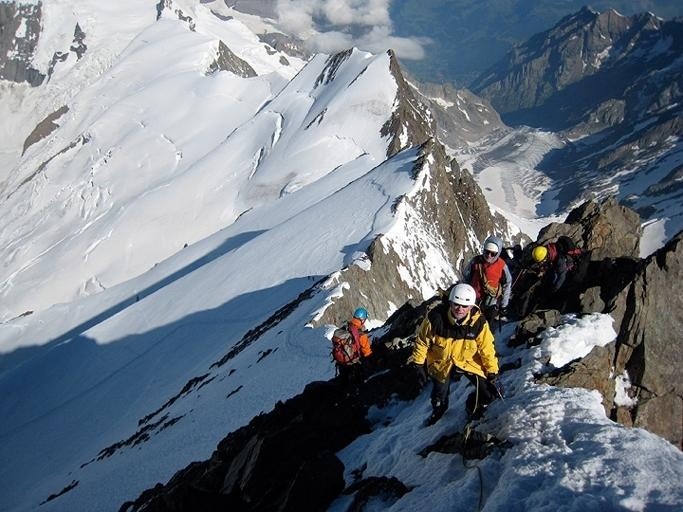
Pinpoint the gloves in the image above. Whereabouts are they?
[486,374,505,399]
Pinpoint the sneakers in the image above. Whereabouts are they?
[423,412,443,427]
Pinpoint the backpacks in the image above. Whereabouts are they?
[329,324,363,367]
[554,235,583,262]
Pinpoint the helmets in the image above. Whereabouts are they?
[353,307,369,319]
[448,283,477,307]
[484,242,499,253]
[531,246,548,264]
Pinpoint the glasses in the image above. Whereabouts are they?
[485,251,497,257]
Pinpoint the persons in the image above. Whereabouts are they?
[412,282,499,427]
[330,307,374,399]
[461,235,513,325]
[531,235,581,296]
